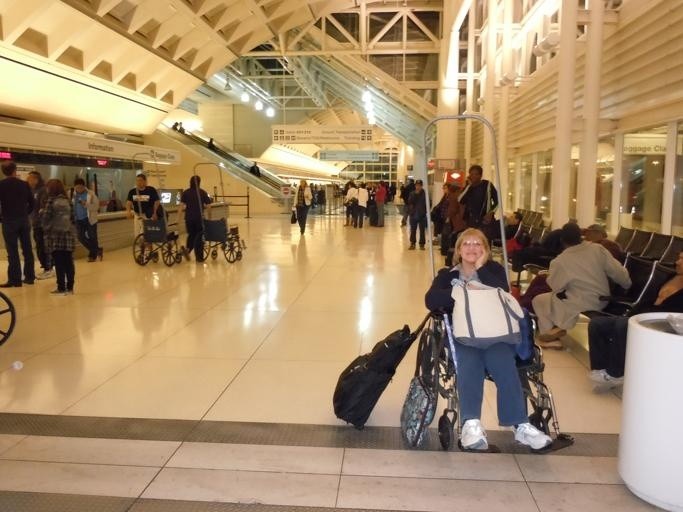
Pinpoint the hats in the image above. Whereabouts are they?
[414,179,423,186]
[584,223,607,238]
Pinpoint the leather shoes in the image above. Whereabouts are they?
[0,282,22,288]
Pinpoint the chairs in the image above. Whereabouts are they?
[481,206,680,357]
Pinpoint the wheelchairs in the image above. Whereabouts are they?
[188,160,248,264]
[127,150,183,267]
[412,111,574,458]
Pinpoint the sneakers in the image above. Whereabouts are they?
[22,280,34,284]
[511,422,553,450]
[539,339,567,351]
[586,368,624,395]
[408,244,415,250]
[87,246,104,262]
[419,245,425,250]
[538,326,567,342]
[48,286,73,297]
[35,272,52,280]
[460,418,488,451]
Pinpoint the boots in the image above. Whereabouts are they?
[344,216,354,226]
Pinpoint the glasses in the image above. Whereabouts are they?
[462,239,482,249]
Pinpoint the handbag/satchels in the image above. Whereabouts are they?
[290,210,297,224]
[451,280,525,350]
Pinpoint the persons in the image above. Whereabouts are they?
[207,136,214,151]
[172,122,177,132]
[579,224,621,259]
[587,250,681,393]
[174,174,213,263]
[25,172,55,280]
[531,221,633,348]
[38,178,76,295]
[249,161,261,178]
[64,177,103,263]
[125,174,159,263]
[291,165,522,257]
[178,122,184,135]
[424,227,553,450]
[0,160,35,288]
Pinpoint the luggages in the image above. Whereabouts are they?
[332,323,418,431]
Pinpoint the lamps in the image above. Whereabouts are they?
[208,64,279,120]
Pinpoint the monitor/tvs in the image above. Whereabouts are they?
[160,192,171,204]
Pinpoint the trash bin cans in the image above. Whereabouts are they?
[370,194,378,225]
[618,312,683,512]
